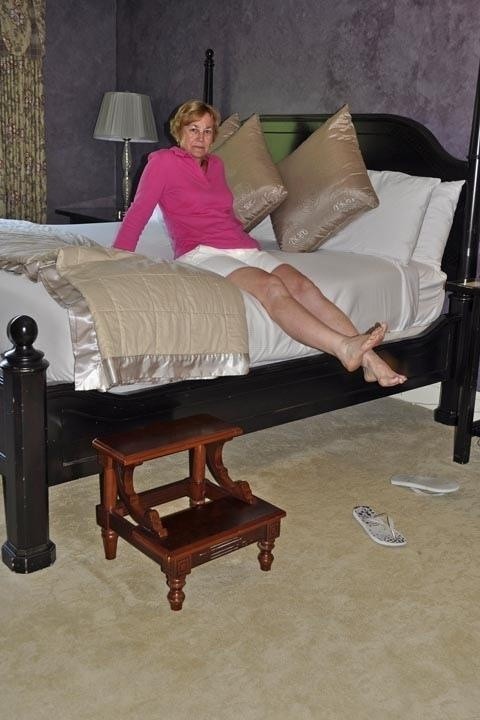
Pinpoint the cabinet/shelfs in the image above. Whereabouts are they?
[91,413,289,611]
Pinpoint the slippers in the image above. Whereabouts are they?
[354,504,407,545]
[391,473,459,496]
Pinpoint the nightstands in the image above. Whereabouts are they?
[443,278,479,466]
[52,192,118,222]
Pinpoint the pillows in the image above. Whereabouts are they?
[207,110,287,234]
[409,178,466,270]
[265,99,380,253]
[207,113,243,155]
[318,160,441,267]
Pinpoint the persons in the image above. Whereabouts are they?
[113,100,407,388]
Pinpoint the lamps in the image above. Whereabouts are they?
[91,89,161,219]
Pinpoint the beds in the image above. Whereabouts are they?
[0,50,480,576]
